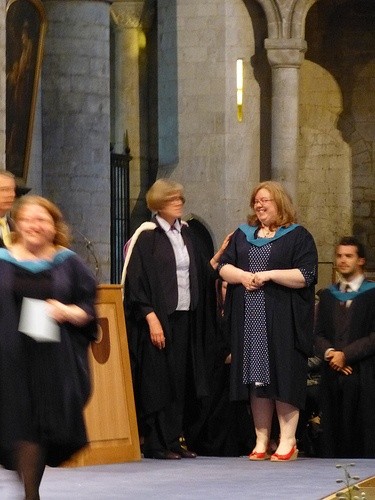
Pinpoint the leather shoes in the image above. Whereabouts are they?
[145,440,197,459]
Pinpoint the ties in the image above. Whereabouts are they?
[1,215,12,249]
[340,281,352,309]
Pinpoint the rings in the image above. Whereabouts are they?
[247,284,250,287]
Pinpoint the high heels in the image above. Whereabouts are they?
[248,444,299,461]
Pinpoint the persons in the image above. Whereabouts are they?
[215,277,252,457]
[299,288,331,457]
[312,236,375,459]
[217,181,319,462]
[0,170,18,249]
[6,19,38,177]
[0,194,98,500]
[120,179,234,459]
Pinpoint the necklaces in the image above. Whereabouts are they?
[261,229,275,238]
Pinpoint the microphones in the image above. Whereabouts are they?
[86,241,99,279]
